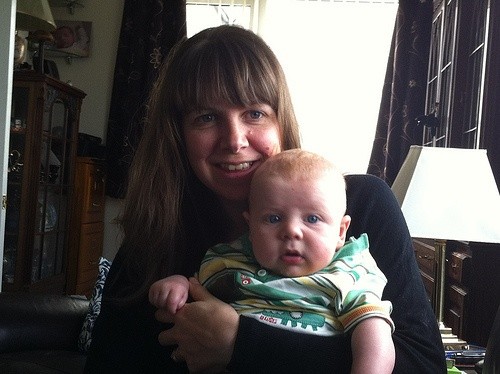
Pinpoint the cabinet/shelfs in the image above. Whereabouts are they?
[65,156,107,302]
[1,71,89,300]
[410,0,500,348]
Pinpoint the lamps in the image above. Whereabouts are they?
[13,0,57,67]
[390,145,500,351]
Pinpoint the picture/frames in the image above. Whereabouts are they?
[43,19,92,58]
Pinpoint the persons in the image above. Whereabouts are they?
[87,25,448,372]
[141,147,396,374]
